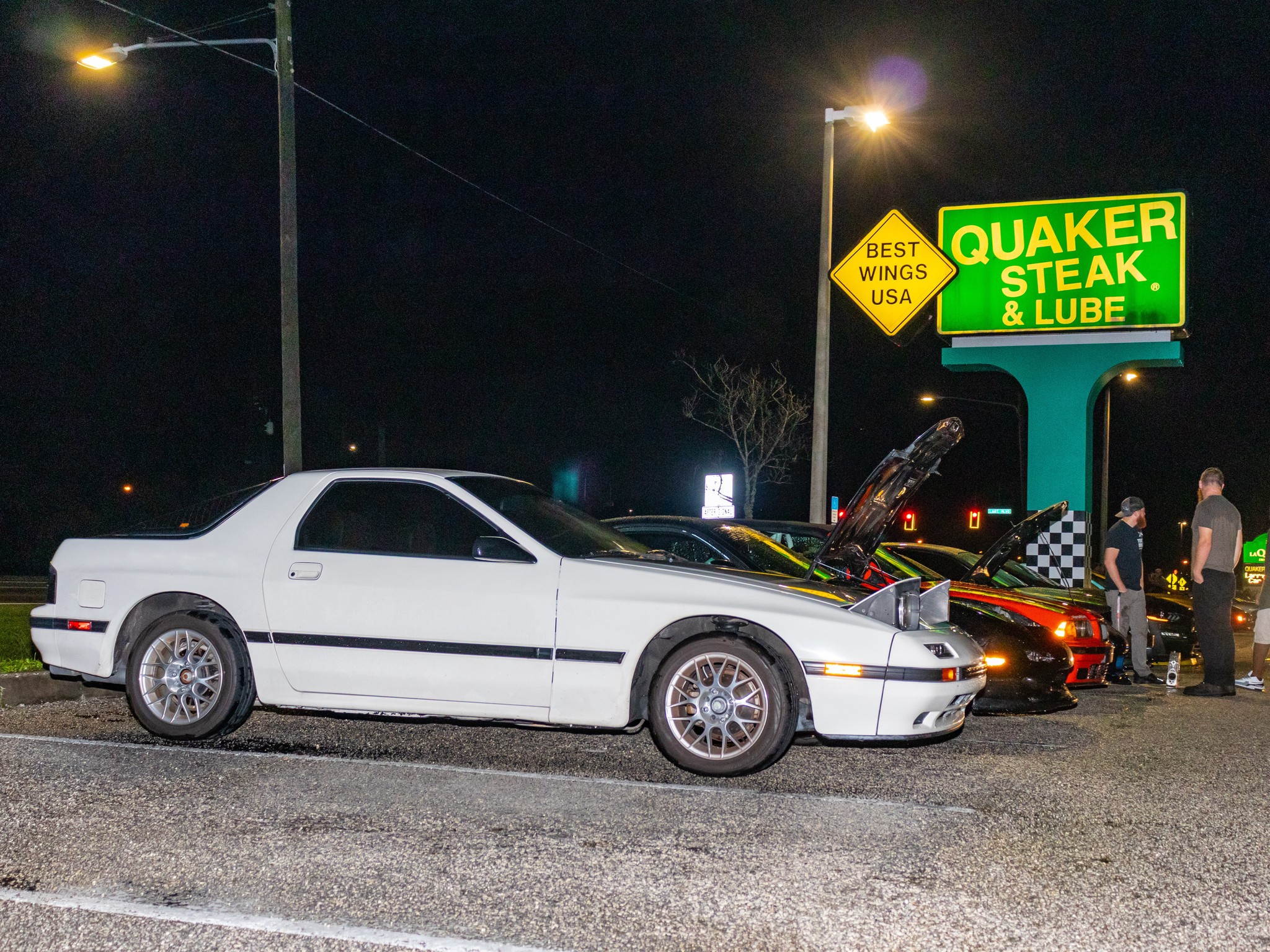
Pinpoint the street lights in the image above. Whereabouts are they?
[76,0,303,470]
[809,105,892,524]
[916,394,1025,519]
[1100,372,1146,566]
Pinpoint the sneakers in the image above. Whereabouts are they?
[1235,670,1266,692]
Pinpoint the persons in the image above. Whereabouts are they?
[1236,527,1270,691]
[1183,466,1243,698]
[1105,494,1168,685]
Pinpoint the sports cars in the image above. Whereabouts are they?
[32,468,985,775]
[597,418,1252,716]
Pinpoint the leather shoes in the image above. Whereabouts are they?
[1133,673,1166,684]
[1111,674,1132,685]
[1183,681,1225,697]
[1224,684,1236,696]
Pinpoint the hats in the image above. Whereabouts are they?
[1115,496,1144,517]
